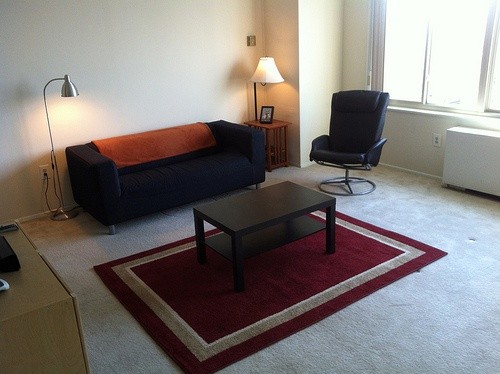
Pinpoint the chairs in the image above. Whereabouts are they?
[309,90,389,196]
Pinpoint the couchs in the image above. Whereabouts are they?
[65,120,266,235]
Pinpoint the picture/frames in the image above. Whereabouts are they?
[260,106,274,124]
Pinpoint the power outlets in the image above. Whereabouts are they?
[40,164,49,179]
[247,36,256,46]
[433,133,442,147]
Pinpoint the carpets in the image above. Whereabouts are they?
[93,207,448,374]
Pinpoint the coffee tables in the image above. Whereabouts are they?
[192,180,337,293]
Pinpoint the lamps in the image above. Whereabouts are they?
[249,56,285,121]
[43,74,80,220]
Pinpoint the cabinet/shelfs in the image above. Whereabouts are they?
[441,127,500,197]
[0,220,91,374]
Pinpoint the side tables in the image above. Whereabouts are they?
[244,118,291,172]
